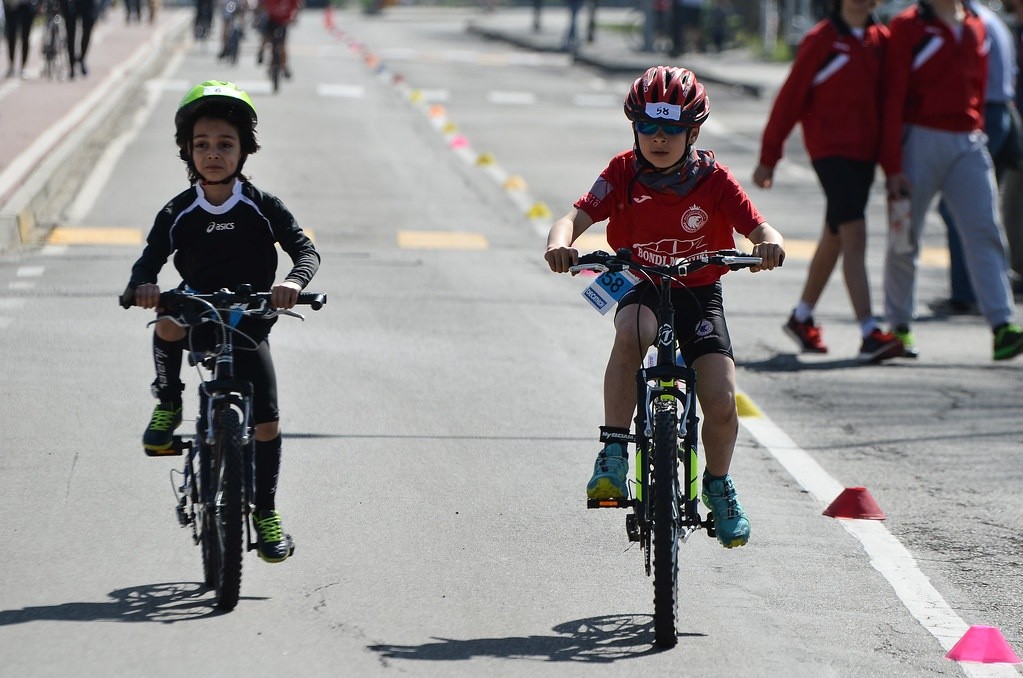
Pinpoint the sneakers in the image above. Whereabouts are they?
[588,443,633,500]
[891,325,918,358]
[783,308,827,353]
[856,328,903,363]
[701,476,749,551]
[991,326,1022,360]
[252,507,291,564]
[142,396,183,448]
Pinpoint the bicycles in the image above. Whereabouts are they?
[117,283,330,616]
[556,246,786,650]
[39,1,289,94]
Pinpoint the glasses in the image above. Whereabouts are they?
[634,121,688,135]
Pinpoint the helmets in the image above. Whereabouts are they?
[624,65,710,127]
[175,80,258,129]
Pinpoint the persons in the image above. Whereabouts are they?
[941,0,1020,314]
[255,0,300,79]
[885,0,1022,361]
[544,64,786,549]
[58,0,100,78]
[149,0,162,27]
[751,0,904,362]
[194,0,216,40]
[3,0,41,79]
[120,78,321,564]
[217,0,252,59]
[651,0,732,57]
[125,0,142,25]
